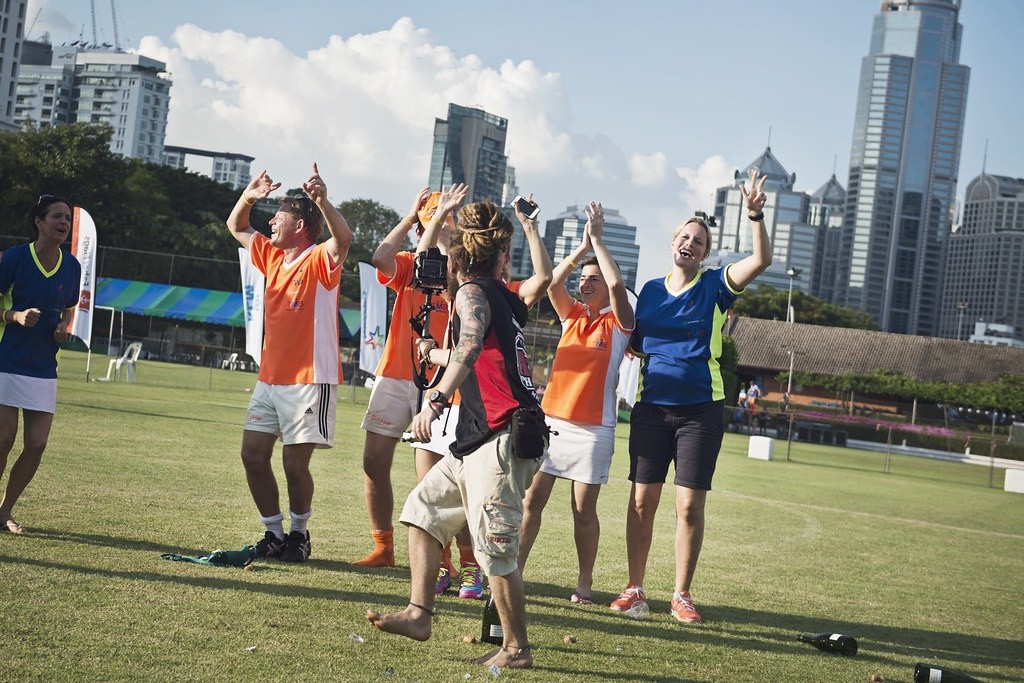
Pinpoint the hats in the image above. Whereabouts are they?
[417,192,456,233]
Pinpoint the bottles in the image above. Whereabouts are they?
[914,663,985,683]
[481,590,504,646]
[796,633,858,657]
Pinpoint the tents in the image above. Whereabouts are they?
[95,277,245,357]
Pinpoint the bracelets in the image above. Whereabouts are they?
[430,215,444,227]
[428,399,444,421]
[243,190,257,206]
[419,345,435,371]
[2,309,11,324]
[748,212,764,220]
[400,215,413,228]
[566,256,578,268]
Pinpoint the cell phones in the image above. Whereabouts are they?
[509,195,541,219]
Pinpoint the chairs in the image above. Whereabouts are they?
[221,354,238,371]
[106,341,142,384]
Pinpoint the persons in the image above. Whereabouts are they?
[356,186,460,579]
[365,183,552,669]
[612,171,772,623]
[0,195,80,535]
[515,201,635,604]
[225,162,352,561]
[733,379,768,437]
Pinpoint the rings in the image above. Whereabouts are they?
[56,329,60,333]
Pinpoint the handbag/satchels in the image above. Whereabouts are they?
[510,406,545,460]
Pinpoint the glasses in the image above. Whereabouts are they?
[36,194,55,206]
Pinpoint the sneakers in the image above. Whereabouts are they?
[457,561,485,599]
[280,529,311,562]
[670,590,701,624]
[610,584,648,611]
[248,531,288,558]
[434,561,453,594]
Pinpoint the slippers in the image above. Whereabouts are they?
[0,520,29,534]
[570,589,594,605]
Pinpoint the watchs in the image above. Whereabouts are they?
[430,390,449,407]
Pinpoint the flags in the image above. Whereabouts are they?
[238,247,265,368]
[59,207,97,348]
[359,261,387,376]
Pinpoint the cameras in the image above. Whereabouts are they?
[415,249,449,291]
